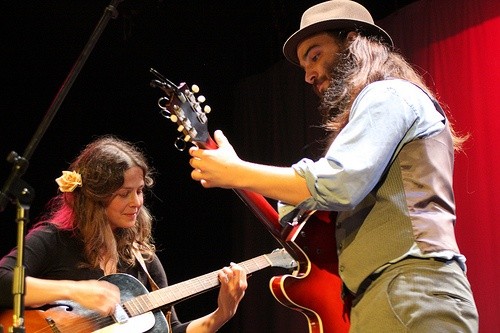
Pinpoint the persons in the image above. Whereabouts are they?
[1,135,250,333]
[188,0,481,331]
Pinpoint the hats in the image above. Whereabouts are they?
[282,0,394,68]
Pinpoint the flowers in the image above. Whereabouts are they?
[54,168,83,193]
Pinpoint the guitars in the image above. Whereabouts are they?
[0,245,298,333]
[145,62,353,332]
